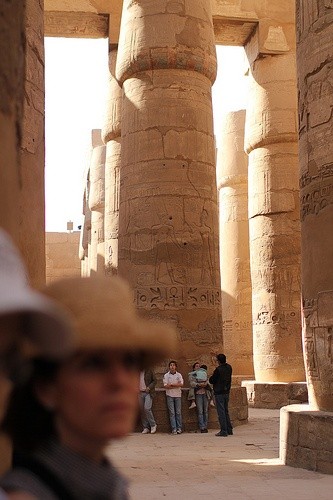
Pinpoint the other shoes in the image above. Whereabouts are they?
[142,428,150,434]
[150,424,157,433]
[189,402,196,408]
[210,400,214,406]
[215,432,232,436]
[201,429,208,433]
[172,429,181,434]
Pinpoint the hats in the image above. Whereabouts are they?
[23,277,175,364]
[1,228,51,313]
